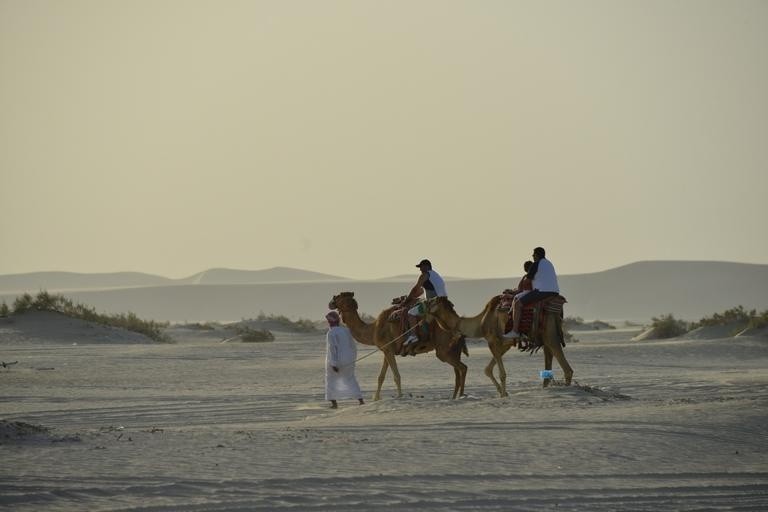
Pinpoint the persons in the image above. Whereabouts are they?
[400,259,448,346]
[325,311,366,409]
[506,260,533,315]
[501,246,560,339]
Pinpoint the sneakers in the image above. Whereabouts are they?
[403,335,419,345]
[503,331,520,338]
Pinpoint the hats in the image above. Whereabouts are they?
[416,260,430,266]
